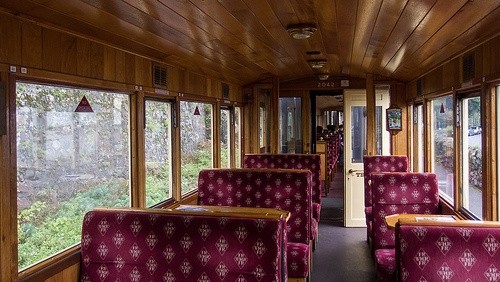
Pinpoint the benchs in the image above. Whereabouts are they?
[77,130,343,282]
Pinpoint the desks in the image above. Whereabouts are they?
[384,213,461,230]
[174,204,291,222]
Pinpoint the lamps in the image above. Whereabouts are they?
[286,25,317,40]
[318,74,329,80]
[308,59,327,68]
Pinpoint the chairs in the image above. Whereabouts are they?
[371,172,441,282]
[395,220,500,282]
[363,155,408,246]
